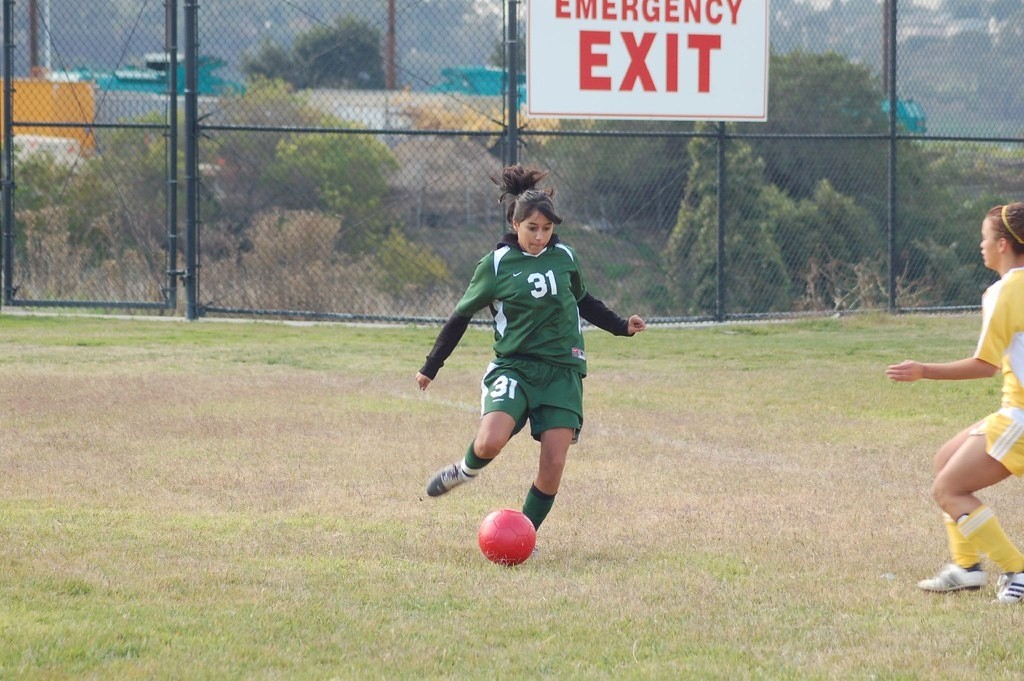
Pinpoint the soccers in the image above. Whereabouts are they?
[478,508,537,567]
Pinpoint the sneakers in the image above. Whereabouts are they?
[427,461,469,496]
[918,563,987,591]
[991,572,1024,605]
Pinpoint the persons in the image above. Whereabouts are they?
[415,163,645,531]
[886,204,1024,609]
